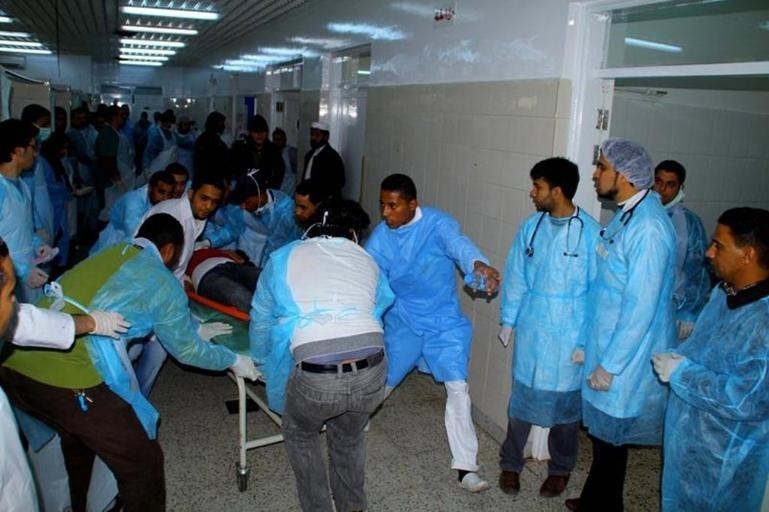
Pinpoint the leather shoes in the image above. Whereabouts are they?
[498,471,520,495]
[540,476,569,498]
[565,498,580,511]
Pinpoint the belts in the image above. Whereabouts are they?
[297,347,385,376]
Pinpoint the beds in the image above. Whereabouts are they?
[181,292,328,496]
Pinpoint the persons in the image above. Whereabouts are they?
[647,160,711,342]
[652,206,769,512]
[364,174,501,492]
[499,157,603,499]
[565,139,679,512]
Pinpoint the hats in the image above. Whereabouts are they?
[157,109,175,123]
[247,115,268,132]
[178,116,195,127]
[311,122,329,131]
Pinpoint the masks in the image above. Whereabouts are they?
[31,124,51,144]
[252,190,274,217]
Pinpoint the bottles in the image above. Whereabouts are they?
[463,273,497,294]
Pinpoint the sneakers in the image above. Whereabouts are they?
[457,472,490,492]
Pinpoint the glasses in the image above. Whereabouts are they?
[25,143,36,152]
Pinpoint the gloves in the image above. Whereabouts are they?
[86,309,130,339]
[229,353,265,382]
[652,352,682,383]
[193,239,210,252]
[196,321,233,342]
